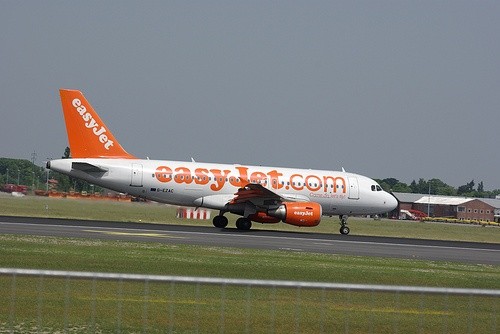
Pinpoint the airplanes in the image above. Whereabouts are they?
[46,89,400,235]
